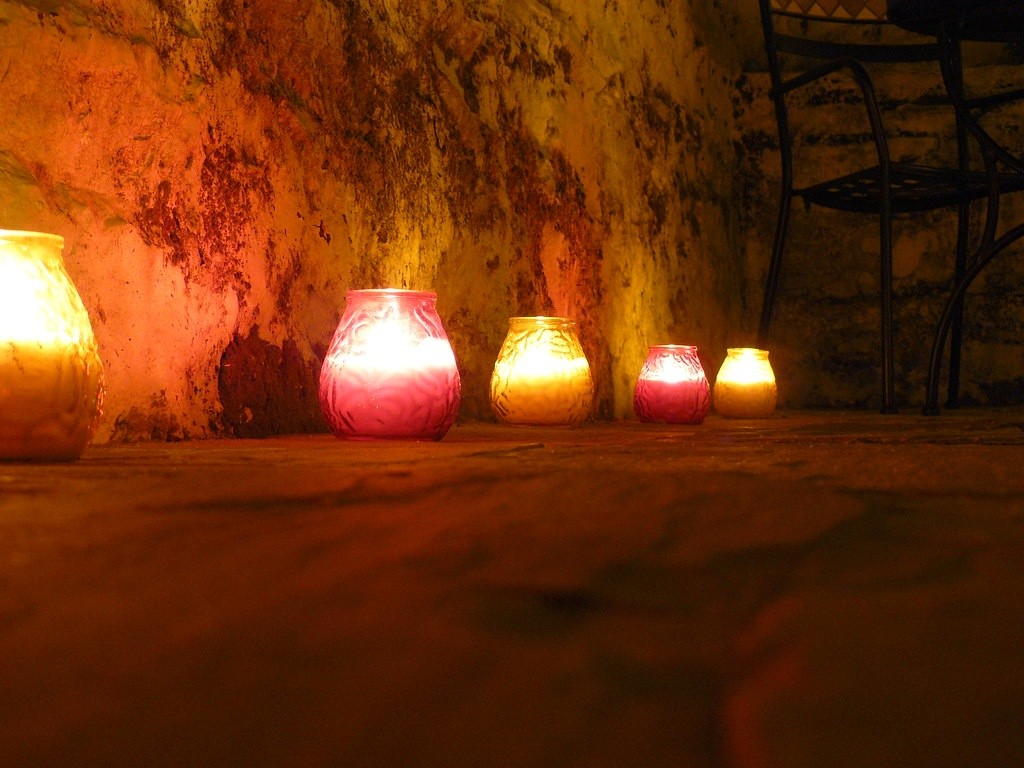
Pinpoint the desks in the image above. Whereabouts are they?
[888,0,1024,415]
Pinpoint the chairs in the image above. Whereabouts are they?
[755,0,1024,416]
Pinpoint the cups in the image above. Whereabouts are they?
[489,317,594,424]
[713,347,776,418]
[634,344,710,425]
[319,289,461,442]
[0,230,105,463]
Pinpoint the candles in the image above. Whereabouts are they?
[488,315,595,430]
[713,347,778,417]
[633,345,711,426]
[0,229,108,464]
[317,288,461,443]
